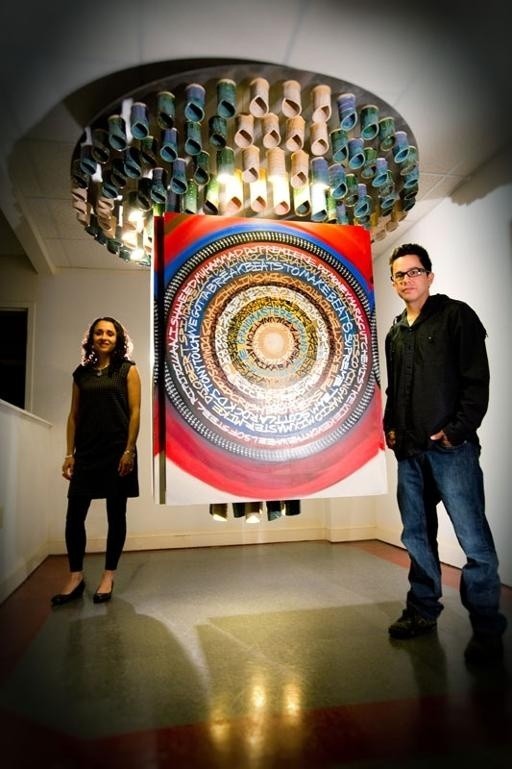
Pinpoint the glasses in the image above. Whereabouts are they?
[393,266,431,283]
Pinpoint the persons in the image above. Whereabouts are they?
[379,244,508,660]
[50,316,142,607]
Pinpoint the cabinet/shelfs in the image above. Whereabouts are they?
[0,397,54,609]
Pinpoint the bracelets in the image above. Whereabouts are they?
[65,453,75,458]
[123,450,138,457]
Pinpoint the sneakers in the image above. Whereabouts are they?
[463,630,504,667]
[387,603,438,640]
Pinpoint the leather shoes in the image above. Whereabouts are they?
[50,580,86,607]
[93,579,114,604]
